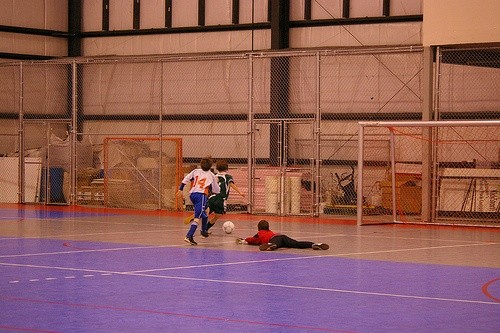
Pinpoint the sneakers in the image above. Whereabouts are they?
[201,231,208,237]
[184,235,197,245]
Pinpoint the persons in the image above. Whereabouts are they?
[236,219,329,251]
[176,157,245,246]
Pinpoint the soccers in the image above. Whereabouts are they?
[222,220,235,234]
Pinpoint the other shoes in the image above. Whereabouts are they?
[200,230,212,234]
[184,214,194,225]
[260,243,277,251]
[312,243,329,250]
